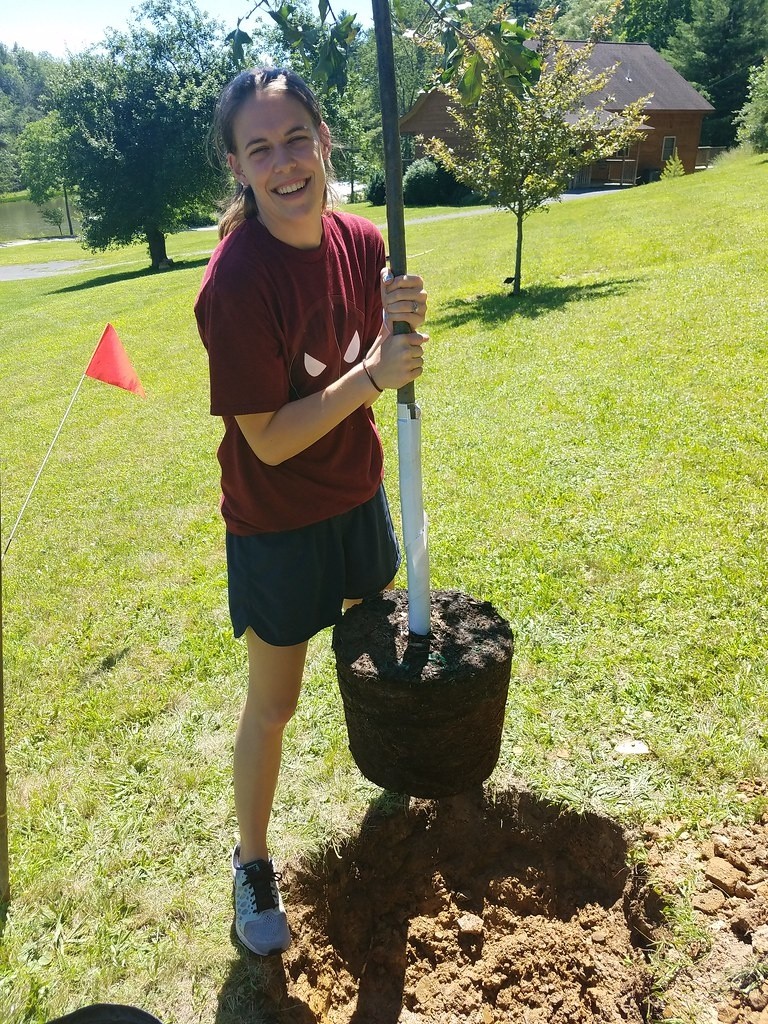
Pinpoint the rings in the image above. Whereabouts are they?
[410,300,418,314]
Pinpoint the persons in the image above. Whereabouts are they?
[191,68,430,955]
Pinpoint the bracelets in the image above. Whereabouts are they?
[361,358,385,394]
[382,308,392,336]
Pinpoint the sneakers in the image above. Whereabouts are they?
[232,841,291,957]
